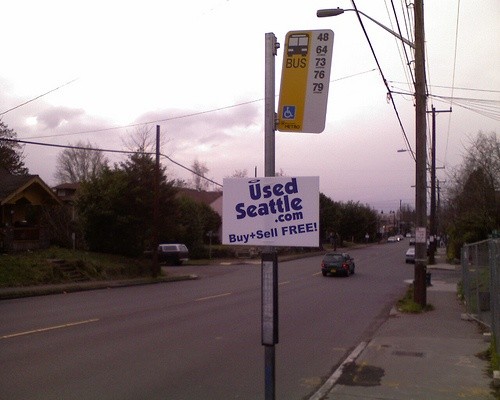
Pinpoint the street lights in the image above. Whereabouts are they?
[395,146,433,177]
[378,208,397,239]
[409,182,438,195]
[316,6,428,307]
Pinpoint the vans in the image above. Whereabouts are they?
[156,242,190,263]
[321,253,354,278]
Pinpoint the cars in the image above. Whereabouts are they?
[405,232,412,239]
[387,233,405,243]
[405,247,416,263]
[409,238,416,245]
